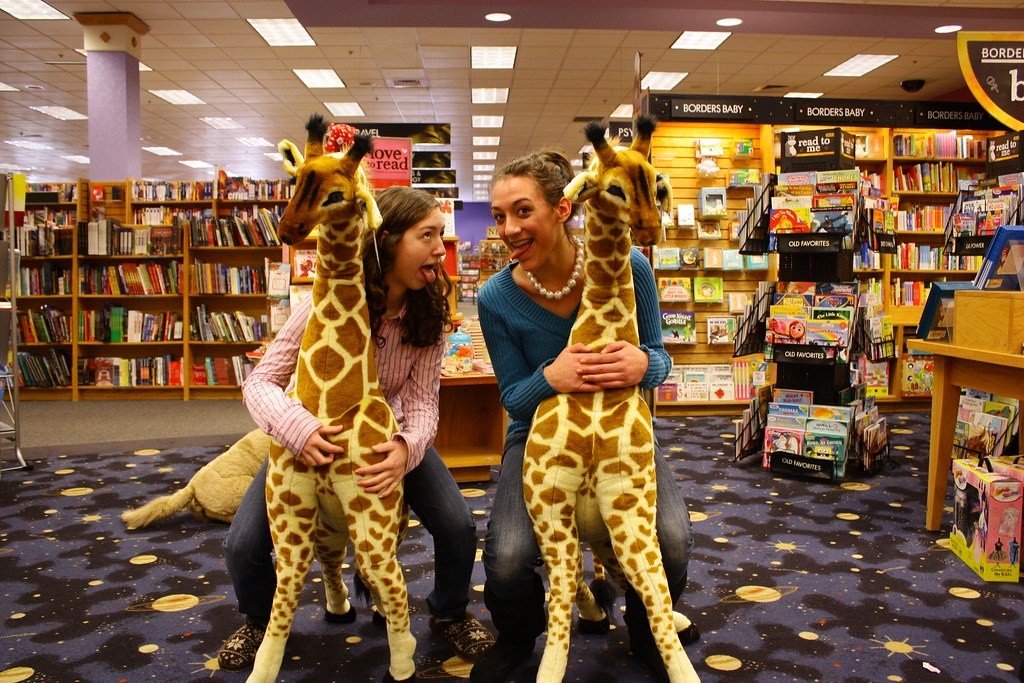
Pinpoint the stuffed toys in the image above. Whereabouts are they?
[522,112,704,683]
[118,427,272,531]
[245,115,418,683]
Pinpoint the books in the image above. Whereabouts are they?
[1,176,318,388]
[652,130,1024,582]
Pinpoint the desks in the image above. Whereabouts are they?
[430,366,506,483]
[903,336,1024,533]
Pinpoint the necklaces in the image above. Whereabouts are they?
[527,236,586,301]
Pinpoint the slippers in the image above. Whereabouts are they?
[218,621,266,670]
[429,612,496,662]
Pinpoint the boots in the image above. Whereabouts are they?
[470,573,545,683]
[622,572,688,683]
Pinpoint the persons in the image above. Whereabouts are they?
[217,187,497,671]
[470,151,699,682]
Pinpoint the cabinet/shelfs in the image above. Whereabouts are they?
[14,229,75,406]
[890,128,1007,402]
[731,130,896,485]
[212,179,295,218]
[24,182,80,226]
[769,127,900,404]
[186,223,287,404]
[128,184,213,222]
[441,237,462,323]
[73,224,184,403]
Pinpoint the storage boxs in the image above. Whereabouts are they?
[947,453,1024,582]
